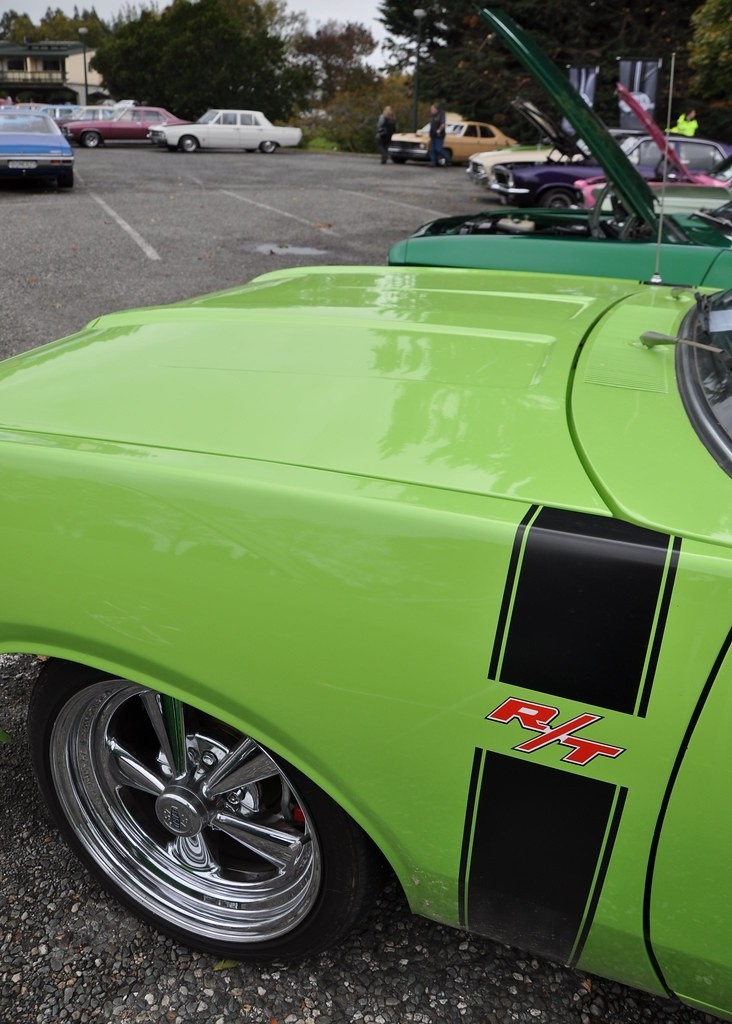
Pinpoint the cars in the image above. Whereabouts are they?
[386,111,521,170]
[59,105,195,148]
[146,108,304,155]
[0,255,732,1024]
[385,3,732,294]
[0,97,141,140]
[464,123,732,208]
[0,108,76,190]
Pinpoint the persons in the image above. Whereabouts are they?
[375,105,398,165]
[664,108,698,137]
[427,102,451,167]
